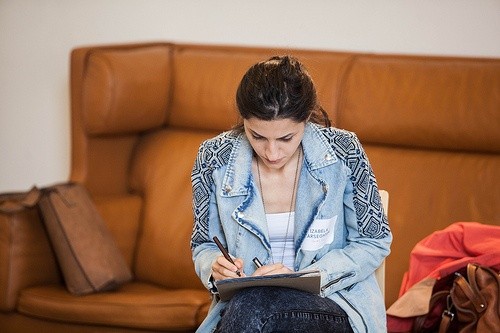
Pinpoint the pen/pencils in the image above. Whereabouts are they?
[213,236,242,278]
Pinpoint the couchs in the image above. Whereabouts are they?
[0,41,500,333]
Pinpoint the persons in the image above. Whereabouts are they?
[191,55,393,333]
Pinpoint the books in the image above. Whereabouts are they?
[216,268,321,301]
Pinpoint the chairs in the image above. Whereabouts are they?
[378,190,390,297]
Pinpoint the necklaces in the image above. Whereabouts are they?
[256,144,302,266]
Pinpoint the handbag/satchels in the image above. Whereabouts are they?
[0,182,132,297]
[414,262,500,333]
